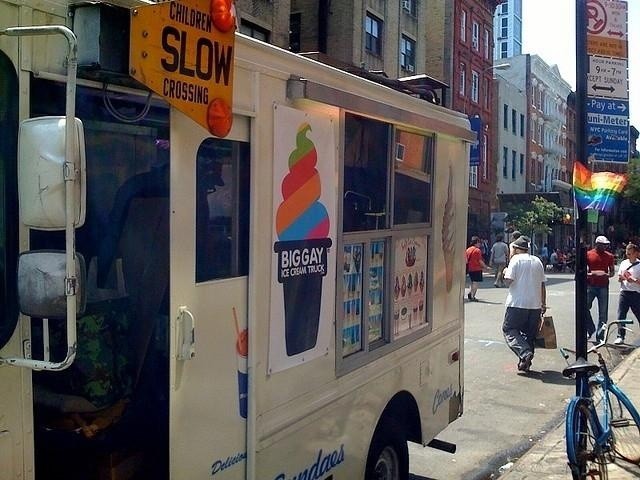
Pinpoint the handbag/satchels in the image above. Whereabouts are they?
[534,317,557,349]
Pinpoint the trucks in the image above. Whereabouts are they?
[0,0,478,480]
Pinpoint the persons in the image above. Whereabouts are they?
[587,236,640,344]
[98,143,224,304]
[465,230,575,302]
[501,235,548,372]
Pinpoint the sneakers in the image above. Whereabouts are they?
[518,352,534,371]
[613,337,624,344]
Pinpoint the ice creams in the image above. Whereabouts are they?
[274,119,332,355]
[394,270,424,299]
[441,162,459,291]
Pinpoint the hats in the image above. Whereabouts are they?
[596,235,611,245]
[513,235,530,250]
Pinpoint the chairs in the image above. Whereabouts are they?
[199,224,231,280]
[343,191,372,232]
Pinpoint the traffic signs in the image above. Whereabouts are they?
[587,55,629,99]
[585,98,632,165]
[586,0,629,58]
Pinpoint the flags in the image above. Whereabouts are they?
[573,160,628,214]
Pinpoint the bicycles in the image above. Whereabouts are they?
[560,318,640,480]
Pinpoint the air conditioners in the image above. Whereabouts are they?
[472,41,477,48]
[402,1,409,12]
[406,64,414,72]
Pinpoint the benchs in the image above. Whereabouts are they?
[548,259,575,273]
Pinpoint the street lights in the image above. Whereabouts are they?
[462,60,511,114]
